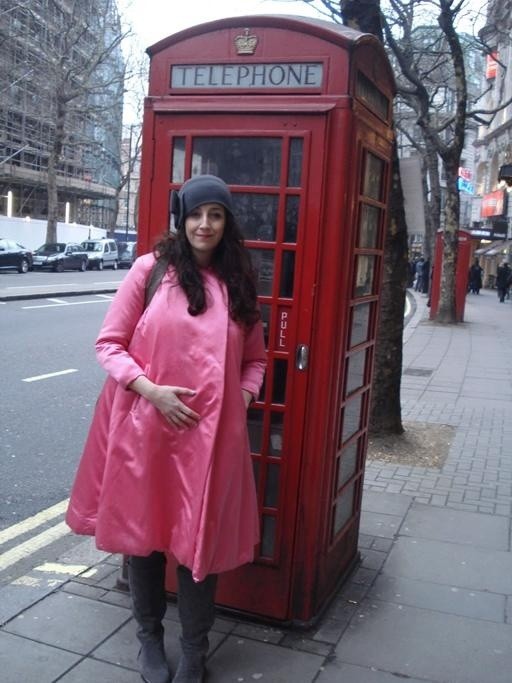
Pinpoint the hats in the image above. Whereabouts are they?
[169,175,235,229]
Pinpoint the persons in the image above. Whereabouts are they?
[406,258,512,302]
[65,175,268,683]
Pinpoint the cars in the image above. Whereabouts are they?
[0,238,33,274]
[117,241,137,270]
[32,242,89,273]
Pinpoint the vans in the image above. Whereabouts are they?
[80,238,119,271]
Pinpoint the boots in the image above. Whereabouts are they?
[128,560,171,682]
[173,565,217,683]
